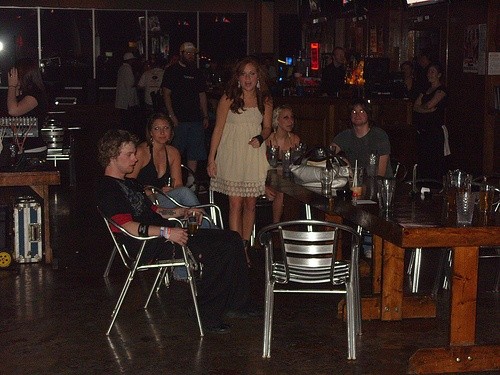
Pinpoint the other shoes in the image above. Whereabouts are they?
[185,181,208,194]
[188,294,247,335]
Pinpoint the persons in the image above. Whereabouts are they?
[97,130,268,326]
[127,116,220,229]
[398,48,450,195]
[134,62,167,116]
[207,59,273,271]
[319,46,350,98]
[115,53,139,133]
[5,57,50,169]
[261,104,311,230]
[161,42,211,159]
[330,102,394,299]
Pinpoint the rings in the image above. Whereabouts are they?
[11,75,13,78]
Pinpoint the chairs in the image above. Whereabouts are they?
[254,221,363,361]
[163,162,202,207]
[95,186,216,338]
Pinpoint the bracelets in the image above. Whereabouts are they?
[7,85,16,87]
[164,226,172,240]
[160,226,165,235]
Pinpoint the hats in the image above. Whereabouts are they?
[180,42,199,53]
[123,52,136,60]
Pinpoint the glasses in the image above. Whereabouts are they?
[351,109,366,114]
[151,125,169,131]
[184,51,196,56]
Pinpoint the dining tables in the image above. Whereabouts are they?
[265,165,500,375]
[0,139,64,266]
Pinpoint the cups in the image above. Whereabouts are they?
[320,168,333,195]
[456,192,476,225]
[268,145,279,167]
[348,167,363,201]
[376,177,396,213]
[447,172,466,209]
[186,212,199,237]
[480,184,494,214]
